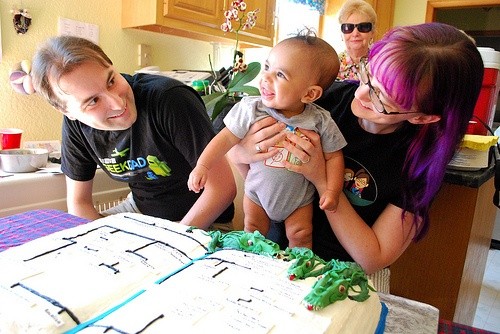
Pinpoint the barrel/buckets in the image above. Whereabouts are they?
[466,46,500,136]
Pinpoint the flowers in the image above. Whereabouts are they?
[197,0,261,124]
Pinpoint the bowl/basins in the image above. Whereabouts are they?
[0,148,50,173]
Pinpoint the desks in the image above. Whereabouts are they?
[390,161,498,325]
[1,209,500,334]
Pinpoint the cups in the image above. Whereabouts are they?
[0,127,23,150]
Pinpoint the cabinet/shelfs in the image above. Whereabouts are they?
[123,0,277,51]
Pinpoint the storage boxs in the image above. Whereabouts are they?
[465,42,500,135]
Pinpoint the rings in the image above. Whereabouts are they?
[255,143,262,153]
[300,156,310,165]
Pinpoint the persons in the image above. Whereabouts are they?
[226,23,484,276]
[333,0,378,81]
[187,36,347,251]
[31,34,237,234]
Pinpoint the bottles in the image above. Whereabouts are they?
[193,79,210,91]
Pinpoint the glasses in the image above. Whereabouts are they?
[340,22,374,34]
[359,55,419,116]
